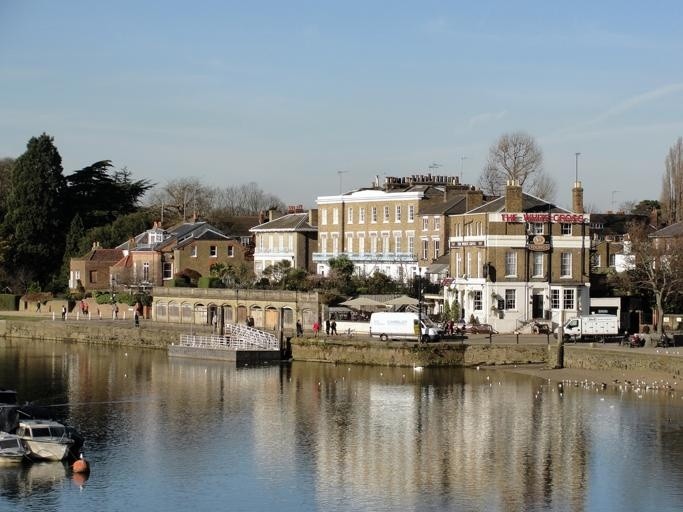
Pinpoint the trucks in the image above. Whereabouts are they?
[554,314,618,342]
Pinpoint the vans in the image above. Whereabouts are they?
[369,311,444,342]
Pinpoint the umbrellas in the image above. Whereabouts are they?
[384,295,432,305]
[338,297,387,315]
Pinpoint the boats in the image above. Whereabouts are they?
[0,391,75,461]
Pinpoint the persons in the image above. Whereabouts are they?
[112,303,119,317]
[660,332,670,347]
[312,321,321,337]
[534,320,542,335]
[326,319,338,336]
[246,316,253,327]
[61,305,66,319]
[212,314,217,331]
[35,300,41,313]
[80,301,88,315]
[296,319,304,338]
[414,319,422,344]
[134,311,139,328]
[447,317,467,337]
[618,330,640,348]
